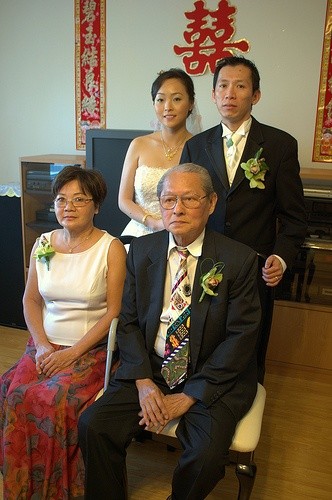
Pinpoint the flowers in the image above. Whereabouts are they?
[240,147,268,189]
[33,240,55,270]
[199,267,222,302]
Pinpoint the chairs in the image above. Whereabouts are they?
[94,236,275,500]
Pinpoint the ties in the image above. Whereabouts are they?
[160,247,192,390]
[226,134,242,174]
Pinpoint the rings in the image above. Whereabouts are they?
[275,277,278,281]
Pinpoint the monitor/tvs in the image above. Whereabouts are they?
[86,129,154,244]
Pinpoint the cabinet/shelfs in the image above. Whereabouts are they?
[19,155,87,286]
[274,178,332,307]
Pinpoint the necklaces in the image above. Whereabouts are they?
[160,130,188,161]
[64,227,94,253]
[225,132,235,147]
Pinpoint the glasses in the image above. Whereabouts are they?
[156,192,209,210]
[52,198,94,208]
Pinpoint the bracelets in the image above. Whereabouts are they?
[142,214,152,224]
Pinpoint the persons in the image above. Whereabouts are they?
[118,68,196,253]
[76,163,263,500]
[179,56,306,384]
[0,164,127,500]
